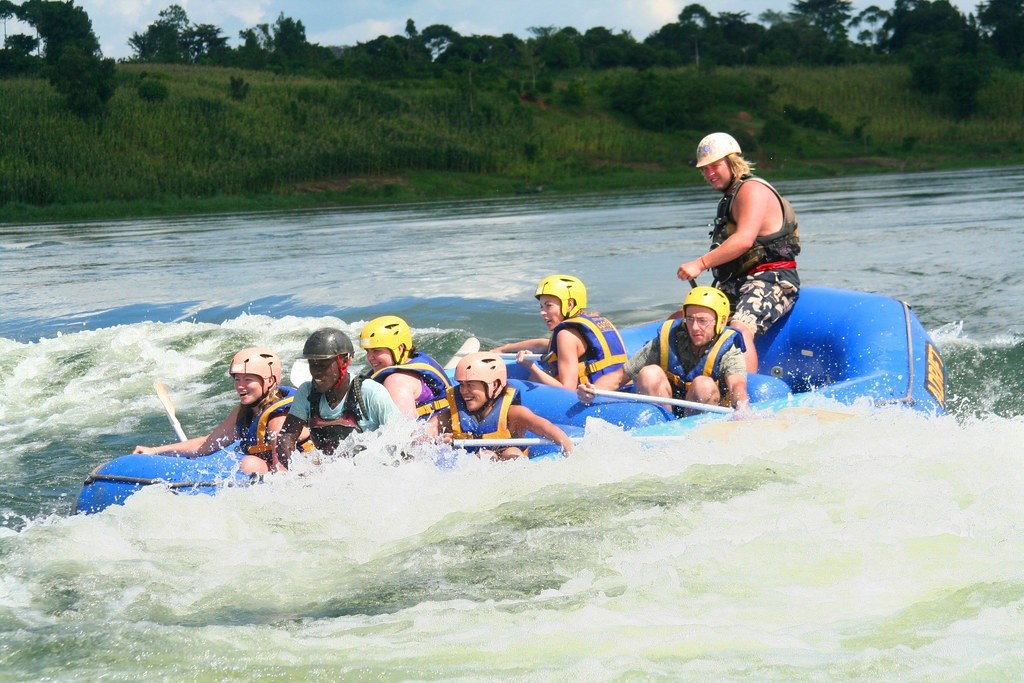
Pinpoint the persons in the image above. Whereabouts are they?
[423,353,572,460]
[359,315,453,438]
[276,328,402,468]
[577,286,752,417]
[491,275,635,392]
[674,132,799,373]
[135,348,318,478]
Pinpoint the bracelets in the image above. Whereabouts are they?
[154,447,158,455]
[701,257,707,268]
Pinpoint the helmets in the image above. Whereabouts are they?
[455,352,508,400]
[535,274,587,318]
[229,347,281,389]
[682,287,730,335]
[295,327,354,363]
[695,131,741,168]
[359,315,413,364]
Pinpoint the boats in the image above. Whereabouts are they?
[70,282,949,521]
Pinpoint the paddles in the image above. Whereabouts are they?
[154,378,188,442]
[442,337,548,370]
[594,388,738,413]
[687,277,698,288]
[452,435,688,445]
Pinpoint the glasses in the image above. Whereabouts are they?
[682,315,715,327]
